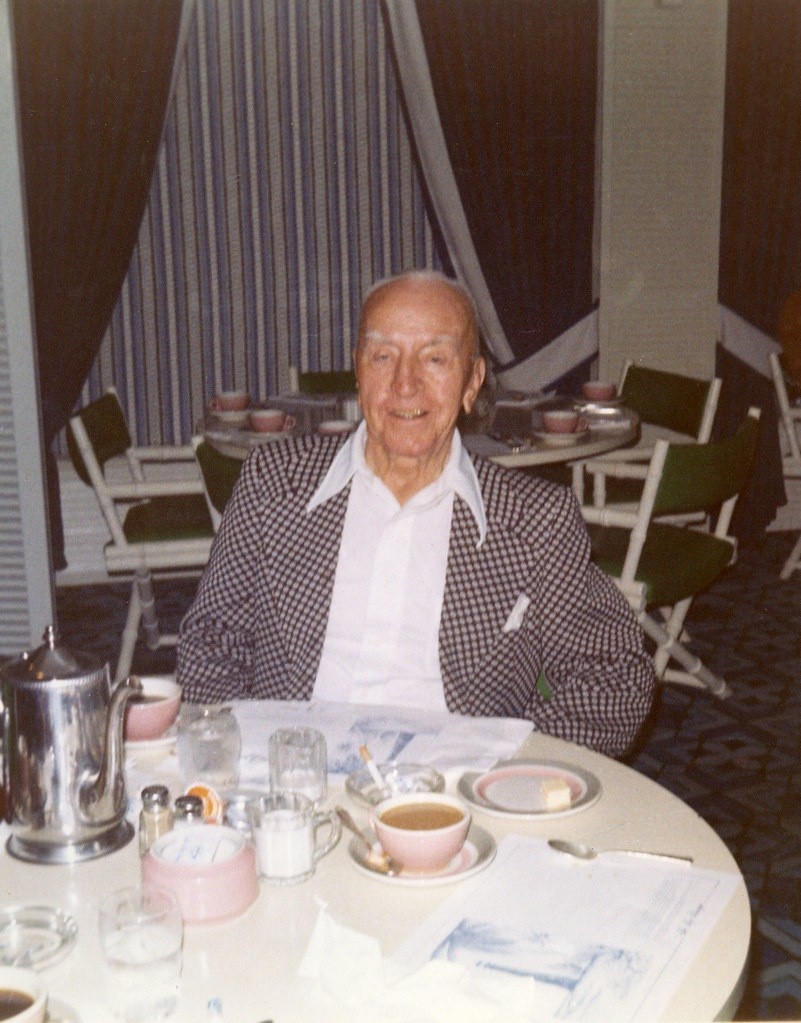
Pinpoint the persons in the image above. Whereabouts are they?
[176,271,659,758]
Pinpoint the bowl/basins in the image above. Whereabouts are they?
[142,827,259,922]
[372,793,472,875]
[0,972,48,1023]
[111,677,183,741]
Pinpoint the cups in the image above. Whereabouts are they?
[245,792,340,887]
[95,888,186,1022]
[583,383,619,402]
[178,711,243,792]
[266,727,327,806]
[208,389,248,411]
[248,410,298,432]
[542,411,587,433]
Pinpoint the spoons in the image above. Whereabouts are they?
[547,839,693,866]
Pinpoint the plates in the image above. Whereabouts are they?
[205,383,626,448]
[458,759,603,817]
[347,824,496,885]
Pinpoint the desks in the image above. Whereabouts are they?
[203,404,641,467]
[0,699,751,1023]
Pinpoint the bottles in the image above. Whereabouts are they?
[139,785,171,846]
[174,798,207,831]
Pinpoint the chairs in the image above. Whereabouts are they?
[62,353,801,698]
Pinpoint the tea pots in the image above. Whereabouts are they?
[0,625,142,865]
